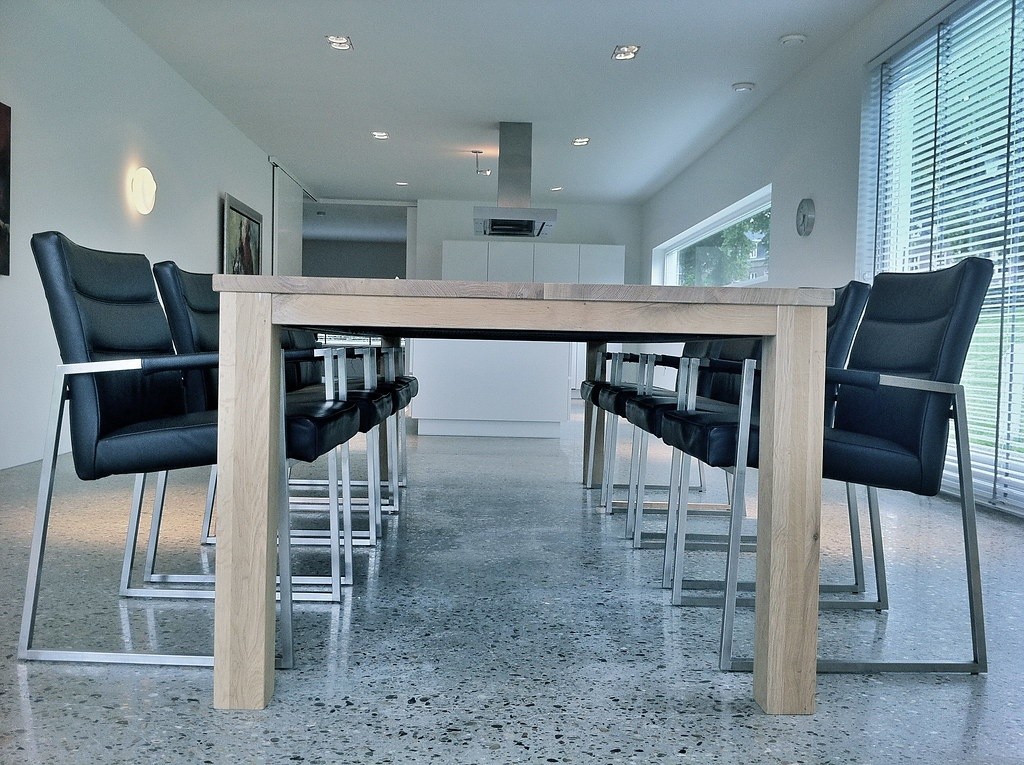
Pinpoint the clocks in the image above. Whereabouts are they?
[794,198,815,239]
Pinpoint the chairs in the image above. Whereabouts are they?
[14,235,296,672]
[201,325,421,549]
[580,331,759,551]
[661,282,868,610]
[665,258,1003,673]
[138,256,373,610]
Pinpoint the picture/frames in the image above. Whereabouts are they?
[220,191,265,275]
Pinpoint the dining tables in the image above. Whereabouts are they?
[205,268,845,715]
